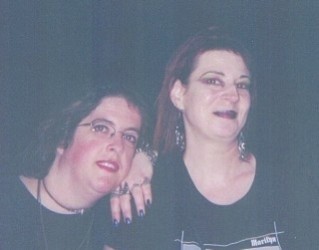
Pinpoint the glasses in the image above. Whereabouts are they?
[76,118,139,145]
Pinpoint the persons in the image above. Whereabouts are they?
[0,76,160,250]
[93,27,319,250]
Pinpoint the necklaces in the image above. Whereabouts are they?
[42,177,94,216]
[35,177,94,250]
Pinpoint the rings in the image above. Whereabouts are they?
[109,188,119,197]
[142,177,150,186]
[132,179,141,187]
[120,182,131,194]
